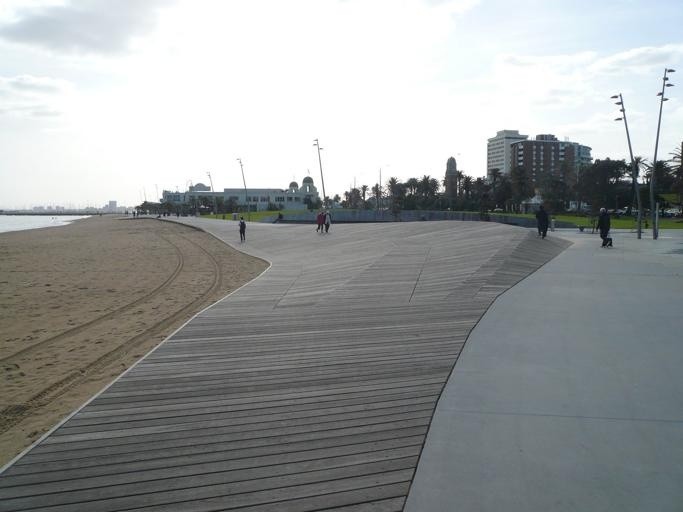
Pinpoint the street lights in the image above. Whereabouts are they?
[313,138,326,198]
[611,92,642,240]
[237,158,248,202]
[650,68,675,239]
[207,172,215,191]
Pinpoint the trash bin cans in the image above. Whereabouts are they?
[551,219,555,232]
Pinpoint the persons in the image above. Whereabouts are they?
[238,215,246,243]
[124,209,179,218]
[270,211,282,224]
[594,207,612,248]
[315,211,331,233]
[549,217,555,233]
[534,205,548,239]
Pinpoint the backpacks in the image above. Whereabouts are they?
[240,222,245,228]
